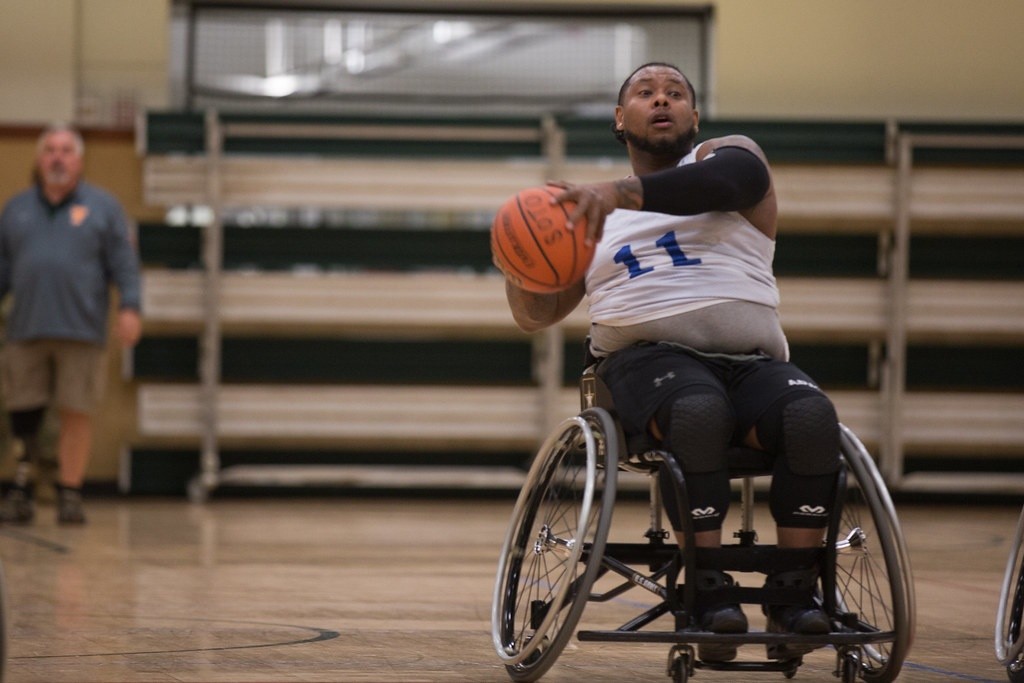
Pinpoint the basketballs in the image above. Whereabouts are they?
[491,184,598,295]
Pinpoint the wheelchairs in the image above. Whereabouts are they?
[492,336,916,683]
[994,499,1024,683]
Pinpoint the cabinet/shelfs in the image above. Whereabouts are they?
[118,99,1024,494]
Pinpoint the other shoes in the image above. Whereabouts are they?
[0,485,32,522]
[694,597,747,662]
[59,489,85,524]
[766,598,829,659]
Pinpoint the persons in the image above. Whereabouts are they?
[499,59,843,665]
[0,125,148,530]
